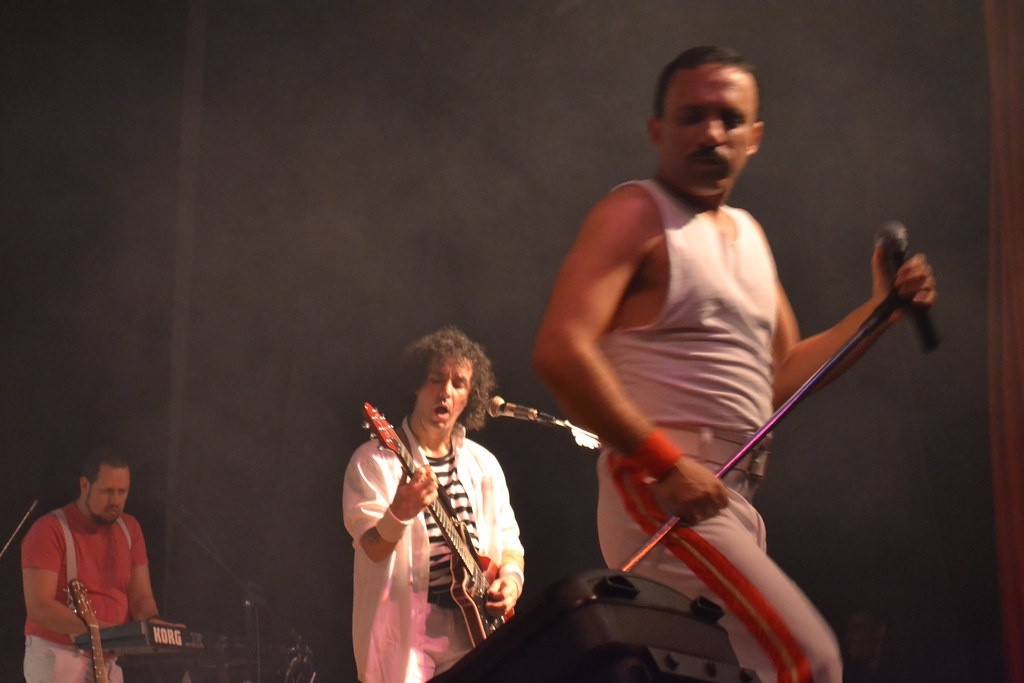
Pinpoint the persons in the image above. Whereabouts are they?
[21,447,161,683]
[530,42,938,682]
[342,329,525,683]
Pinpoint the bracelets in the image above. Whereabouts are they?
[505,574,521,601]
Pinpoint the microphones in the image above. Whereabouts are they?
[874,222,944,353]
[488,395,556,422]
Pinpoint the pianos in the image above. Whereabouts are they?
[69,617,255,682]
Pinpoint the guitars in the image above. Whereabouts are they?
[362,405,515,649]
[63,578,108,683]
